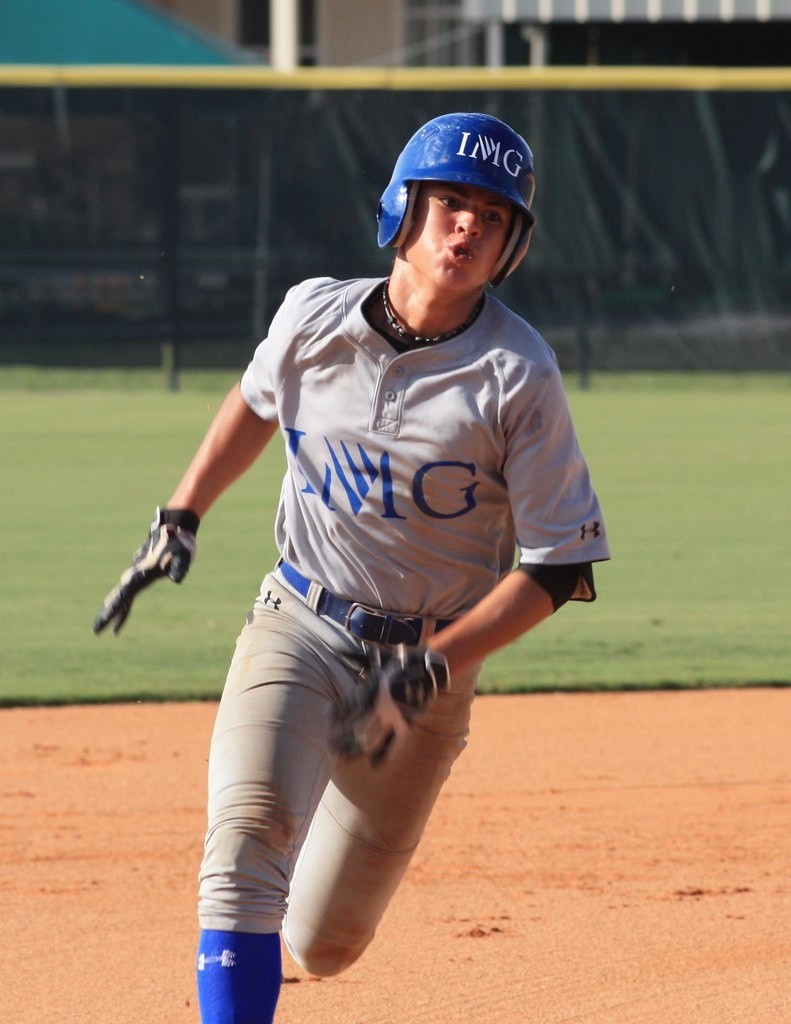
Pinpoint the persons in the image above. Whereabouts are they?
[93,111,611,1024]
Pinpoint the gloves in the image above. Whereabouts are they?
[92,508,201,635]
[325,643,452,771]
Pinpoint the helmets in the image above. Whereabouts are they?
[377,111,536,288]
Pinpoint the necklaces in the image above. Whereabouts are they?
[382,278,483,343]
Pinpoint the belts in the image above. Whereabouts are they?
[277,559,457,647]
